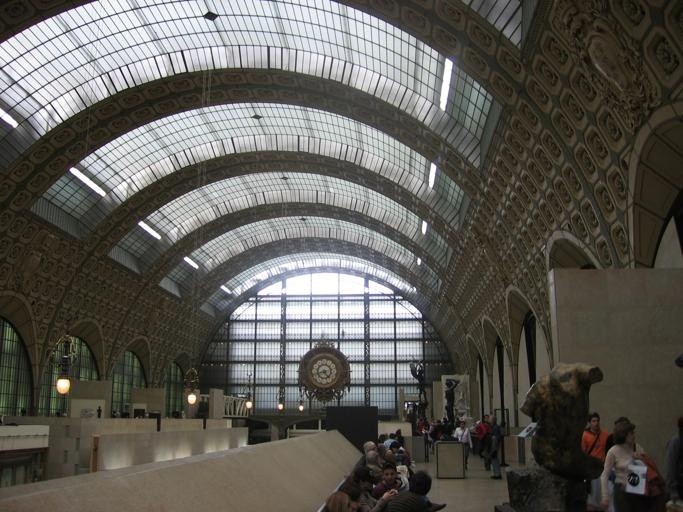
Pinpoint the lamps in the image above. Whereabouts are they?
[183,371,202,405]
[55,340,72,417]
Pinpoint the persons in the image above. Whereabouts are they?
[581,412,683,512]
[454,415,502,480]
[327,417,451,512]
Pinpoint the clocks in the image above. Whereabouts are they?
[296,335,353,405]
